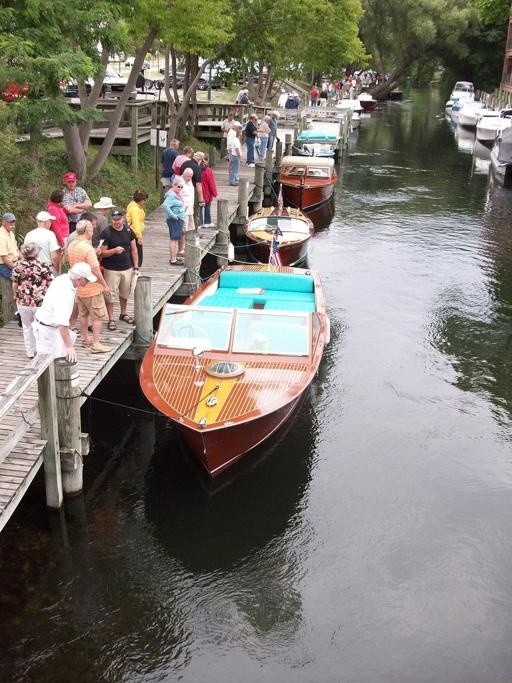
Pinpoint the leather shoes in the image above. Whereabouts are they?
[170,260,184,266]
[169,257,183,261]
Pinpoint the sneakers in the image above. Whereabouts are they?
[82,336,94,347]
[91,342,113,354]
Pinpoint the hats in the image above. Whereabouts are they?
[110,210,124,216]
[94,197,117,208]
[233,121,242,127]
[2,213,16,222]
[72,262,98,284]
[64,172,78,181]
[36,210,57,221]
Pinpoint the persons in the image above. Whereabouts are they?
[180,151,205,240]
[176,167,195,258]
[68,212,98,338]
[159,138,181,186]
[97,211,139,330]
[20,211,61,278]
[10,243,54,358]
[92,197,117,248]
[0,213,24,280]
[61,172,93,232]
[220,69,384,187]
[125,188,149,297]
[160,175,188,266]
[200,152,219,229]
[47,188,68,273]
[66,220,112,352]
[31,261,97,388]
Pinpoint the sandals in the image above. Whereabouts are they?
[108,320,117,330]
[118,314,134,324]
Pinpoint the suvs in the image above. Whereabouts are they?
[65,63,137,102]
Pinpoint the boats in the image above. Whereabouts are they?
[141,265,329,478]
[244,206,313,266]
[492,129,512,188]
[477,109,512,148]
[277,155,337,210]
[454,104,500,127]
[297,101,362,158]
[449,82,476,105]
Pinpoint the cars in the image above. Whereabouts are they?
[169,63,233,91]
[125,58,149,70]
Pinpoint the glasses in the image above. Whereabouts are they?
[177,185,184,189]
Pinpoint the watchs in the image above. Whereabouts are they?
[133,267,139,271]
